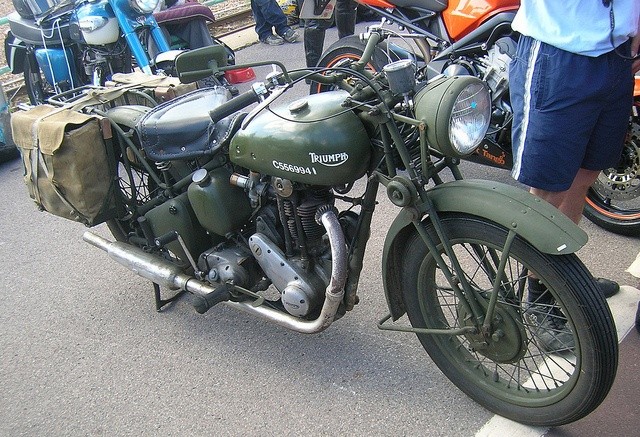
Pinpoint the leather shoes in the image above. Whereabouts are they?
[219,75,238,94]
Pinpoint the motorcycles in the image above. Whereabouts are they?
[310,0,640,236]
[48,34,619,427]
[8,0,171,106]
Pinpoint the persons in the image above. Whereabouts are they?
[250,1,300,46]
[297,0,360,84]
[508,1,640,354]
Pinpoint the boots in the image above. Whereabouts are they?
[335,13,357,39]
[304,26,325,84]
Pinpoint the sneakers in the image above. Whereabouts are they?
[525,303,575,353]
[264,33,284,46]
[595,276,619,297]
[282,27,300,42]
